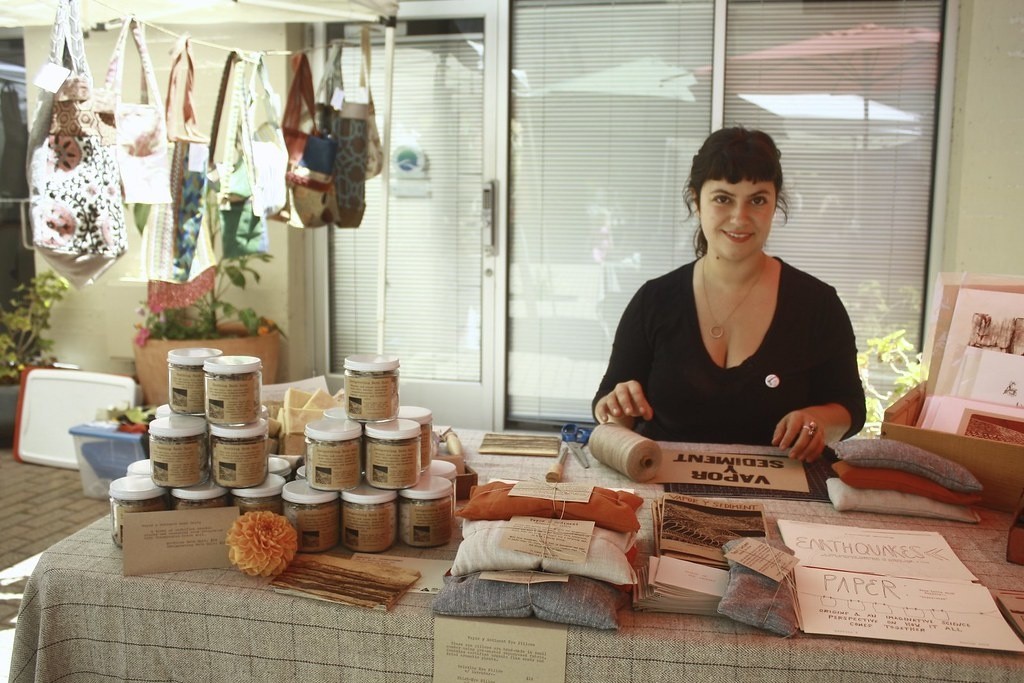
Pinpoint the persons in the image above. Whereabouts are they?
[589,125,874,465]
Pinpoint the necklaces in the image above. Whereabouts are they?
[693,251,771,341]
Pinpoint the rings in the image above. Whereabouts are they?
[802,420,818,438]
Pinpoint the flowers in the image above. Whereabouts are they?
[131,196,289,350]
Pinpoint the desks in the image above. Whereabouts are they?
[4,424,1022,681]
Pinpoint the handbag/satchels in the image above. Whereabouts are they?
[360,27,383,181]
[211,50,273,258]
[141,36,217,313]
[267,53,338,229]
[28,0,129,287]
[103,14,173,205]
[314,44,366,229]
[249,56,290,223]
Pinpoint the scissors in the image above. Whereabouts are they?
[561,422,591,469]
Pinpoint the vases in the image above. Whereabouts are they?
[133,321,282,413]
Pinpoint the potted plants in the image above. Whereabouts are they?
[1,267,72,447]
